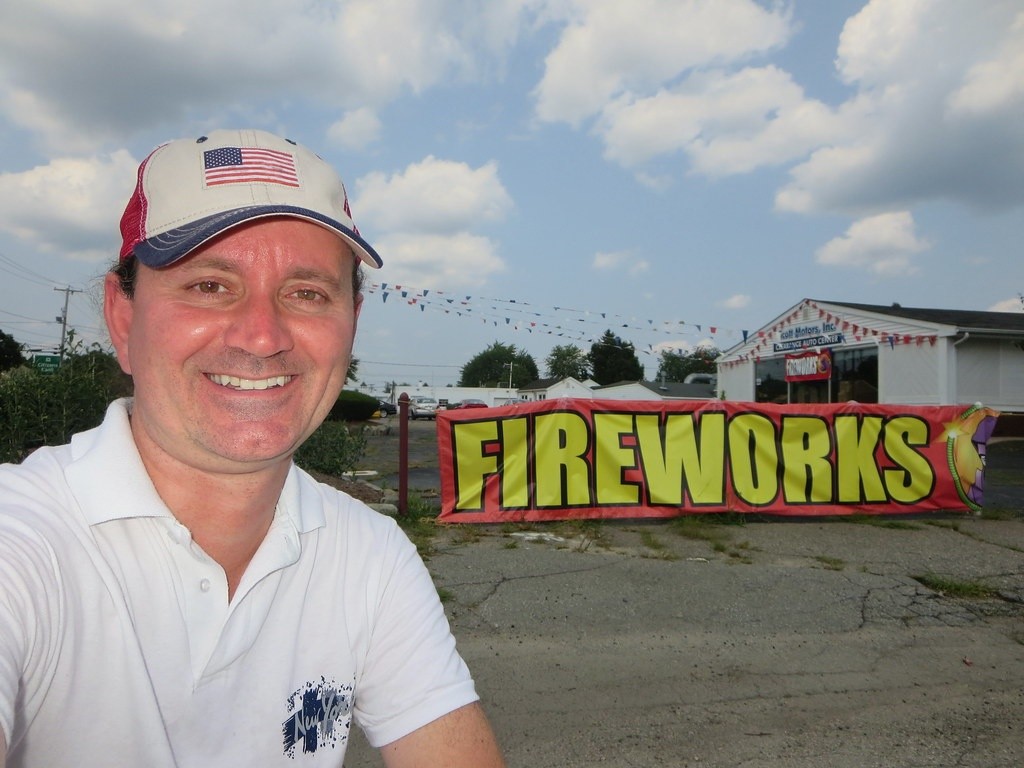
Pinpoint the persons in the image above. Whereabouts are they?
[1,129,505,768]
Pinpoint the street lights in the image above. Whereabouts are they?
[503,362,518,388]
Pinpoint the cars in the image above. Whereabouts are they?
[457,399,488,409]
[408,398,441,420]
[379,400,397,418]
[500,399,526,406]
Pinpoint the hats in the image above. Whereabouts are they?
[119,128,383,270]
